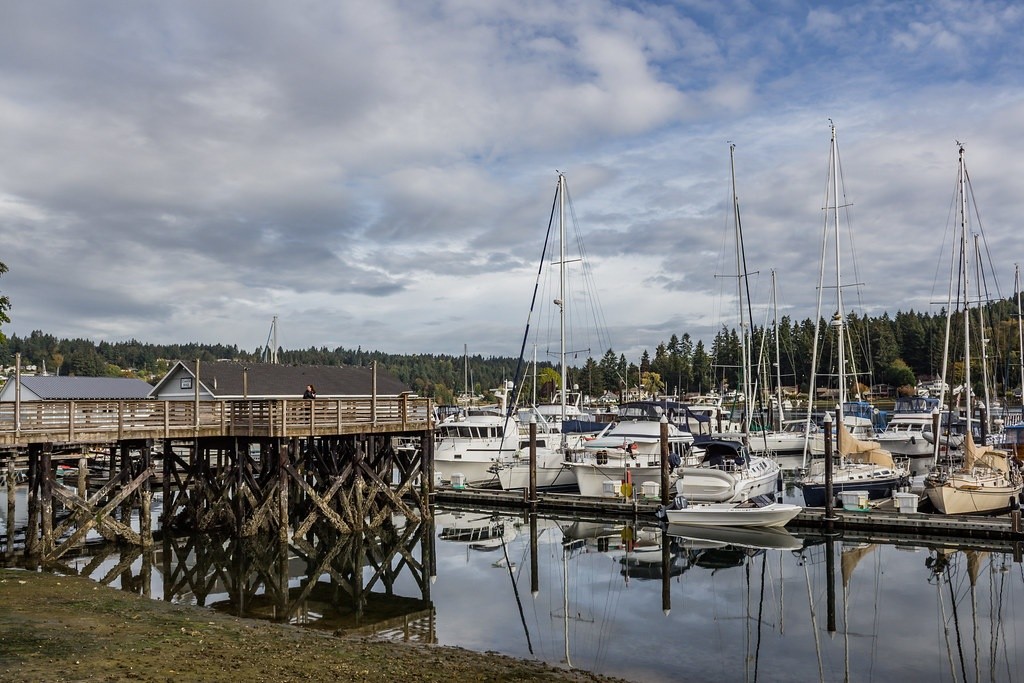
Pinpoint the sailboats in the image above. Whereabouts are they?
[680,140,780,503]
[432,493,1024,683]
[440,172,1015,486]
[800,116,911,506]
[925,142,1021,510]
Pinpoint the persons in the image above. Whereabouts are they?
[303,385,316,424]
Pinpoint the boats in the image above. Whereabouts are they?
[665,503,808,527]
[667,521,805,551]
